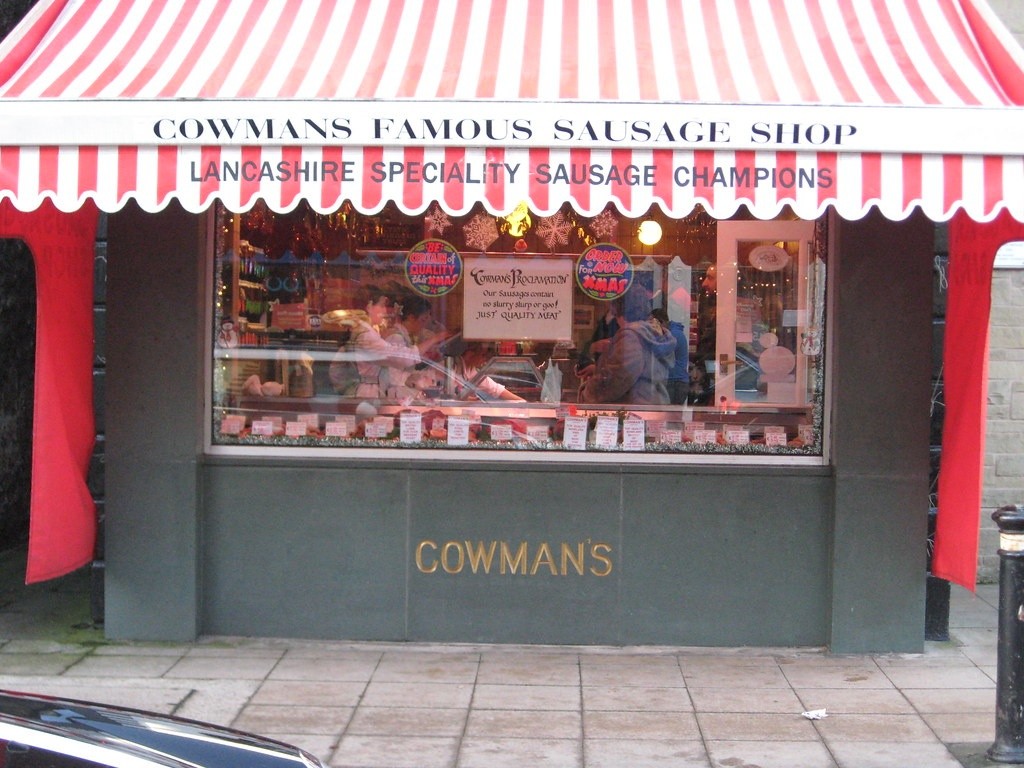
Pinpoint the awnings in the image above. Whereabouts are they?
[0,0,1024,227]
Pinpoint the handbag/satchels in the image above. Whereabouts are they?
[539,358,563,406]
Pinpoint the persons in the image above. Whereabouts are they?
[692,260,769,406]
[324,284,524,402]
[649,307,691,405]
[576,281,678,405]
